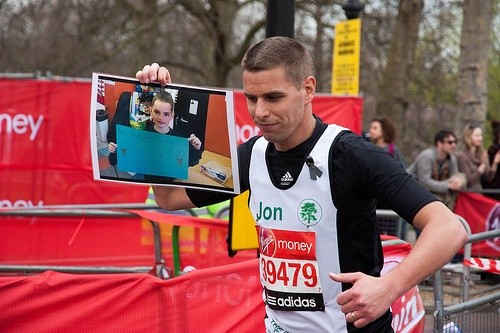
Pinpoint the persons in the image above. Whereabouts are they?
[136,35,469,332]
[365,118,404,172]
[406,130,466,211]
[457,122,491,194]
[108,91,202,183]
[481,120,500,202]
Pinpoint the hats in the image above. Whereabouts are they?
[138,91,154,101]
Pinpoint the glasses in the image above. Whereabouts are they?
[442,140,457,144]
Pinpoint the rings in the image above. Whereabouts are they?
[351,312,356,321]
